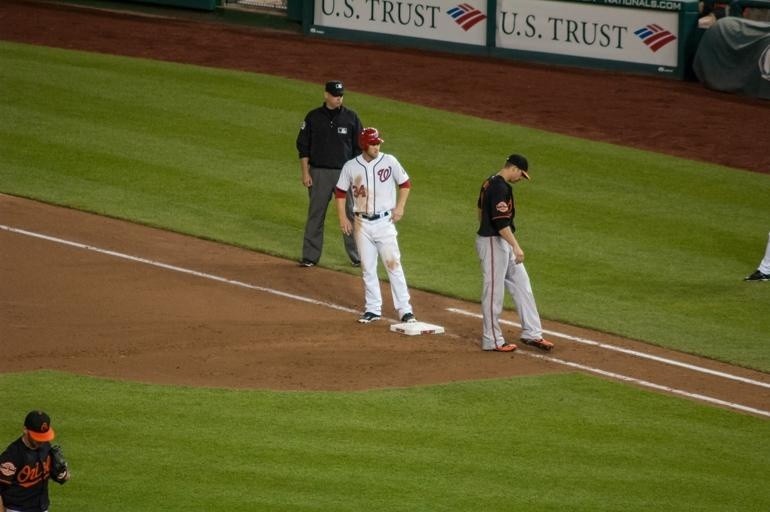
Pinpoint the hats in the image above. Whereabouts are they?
[25,410,54,442]
[326,80,344,96]
[507,155,530,180]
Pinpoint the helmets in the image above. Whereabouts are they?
[358,127,384,151]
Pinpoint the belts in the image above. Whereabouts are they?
[355,212,388,220]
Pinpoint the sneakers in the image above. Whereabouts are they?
[359,312,380,323]
[521,337,554,350]
[403,313,416,322]
[496,343,517,352]
[301,259,313,267]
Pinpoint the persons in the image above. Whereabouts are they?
[0,410,70,510]
[333,127,417,326]
[743,233,769,281]
[297,80,366,269]
[472,154,555,351]
